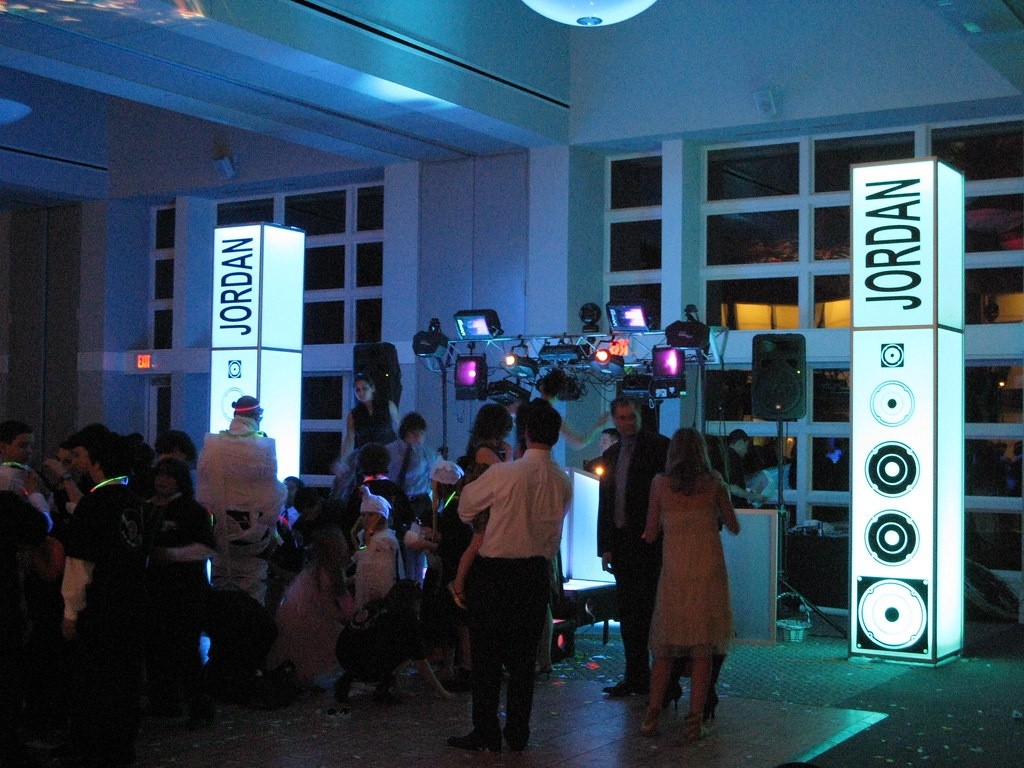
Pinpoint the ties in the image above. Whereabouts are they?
[396,444,412,488]
[612,440,631,530]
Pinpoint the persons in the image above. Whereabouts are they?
[995,441,1022,496]
[0,376,553,731]
[596,396,670,695]
[0,466,52,768]
[641,429,740,739]
[63,434,149,768]
[585,429,620,476]
[447,398,572,752]
[723,429,750,509]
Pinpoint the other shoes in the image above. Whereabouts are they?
[640,720,657,736]
[687,717,701,740]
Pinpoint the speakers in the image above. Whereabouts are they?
[353,342,402,412]
[753,333,806,420]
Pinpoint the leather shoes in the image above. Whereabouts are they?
[447,581,465,610]
[502,727,526,752]
[601,678,650,696]
[448,730,502,753]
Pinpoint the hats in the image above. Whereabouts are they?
[430,462,465,486]
[234,396,264,421]
[360,486,393,519]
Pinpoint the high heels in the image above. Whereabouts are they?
[650,683,683,711]
[685,691,719,720]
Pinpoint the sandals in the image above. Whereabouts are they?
[535,663,554,681]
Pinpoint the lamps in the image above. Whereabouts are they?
[413,299,711,406]
[522,0,656,28]
